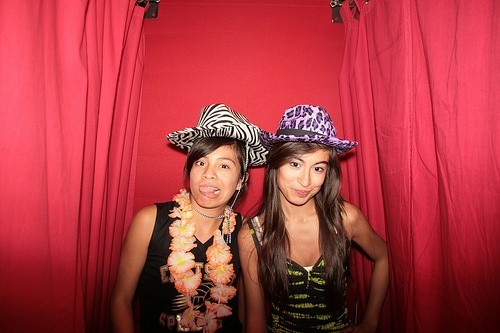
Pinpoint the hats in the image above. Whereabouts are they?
[165,103,269,168]
[258,103,359,154]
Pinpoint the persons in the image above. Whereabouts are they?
[110,102,269,333]
[236,103,390,333]
